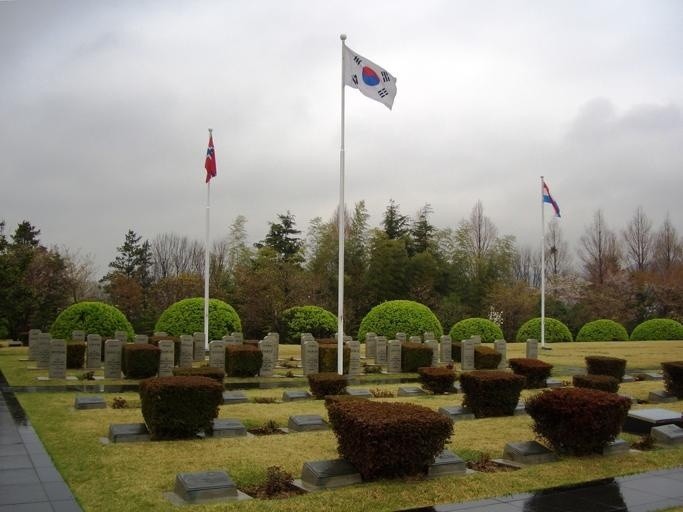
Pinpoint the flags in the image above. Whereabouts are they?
[543,182,561,218]
[343,45,397,110]
[204,137,216,184]
